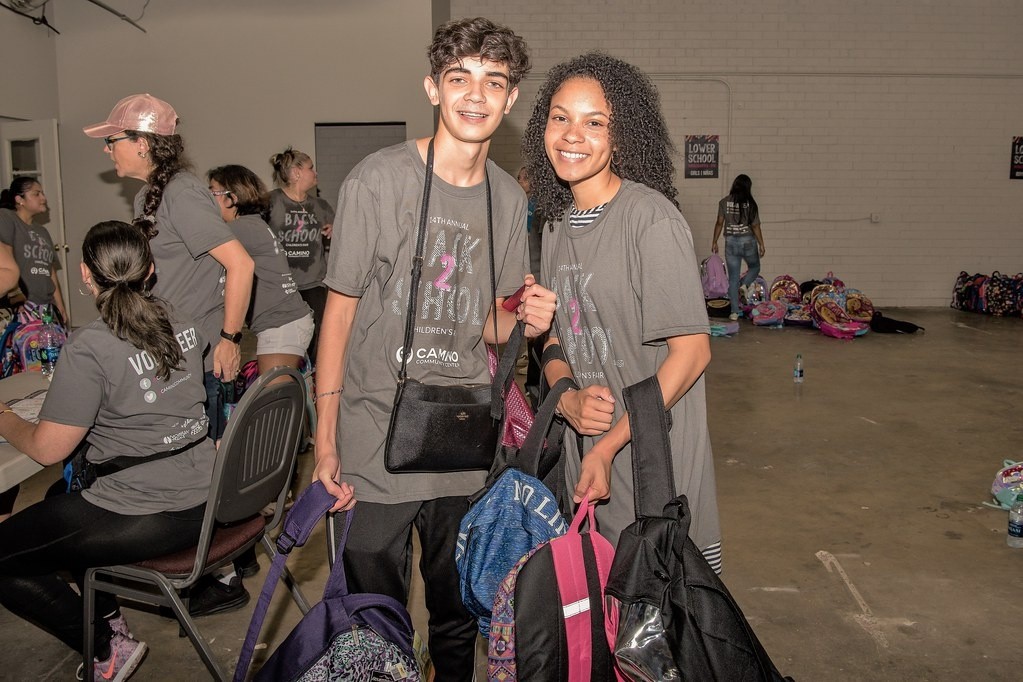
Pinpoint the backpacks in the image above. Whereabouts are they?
[234,479,437,682]
[951,270,1023,319]
[982,458,1023,510]
[0,299,68,379]
[232,359,259,398]
[699,255,875,342]
[454,343,794,682]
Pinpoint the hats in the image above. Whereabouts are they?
[83,94,180,138]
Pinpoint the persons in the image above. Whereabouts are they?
[520,53,723,585]
[83,94,261,619]
[0,221,219,682]
[205,165,315,514]
[712,174,765,321]
[311,17,558,682]
[262,148,335,367]
[0,174,68,324]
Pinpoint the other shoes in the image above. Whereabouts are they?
[730,311,741,320]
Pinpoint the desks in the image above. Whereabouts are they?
[0,370,52,496]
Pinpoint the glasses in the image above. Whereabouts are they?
[105,135,151,151]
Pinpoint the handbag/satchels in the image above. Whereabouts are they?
[58,446,95,490]
[384,375,502,473]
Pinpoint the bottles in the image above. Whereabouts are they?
[794,354,804,383]
[758,286,765,301]
[39,316,60,375]
[1007,495,1023,548]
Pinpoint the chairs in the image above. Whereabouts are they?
[83,365,313,682]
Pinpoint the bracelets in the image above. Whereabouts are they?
[313,388,342,404]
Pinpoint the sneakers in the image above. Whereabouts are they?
[106,614,134,638]
[234,543,260,578]
[159,567,250,616]
[76,632,147,682]
